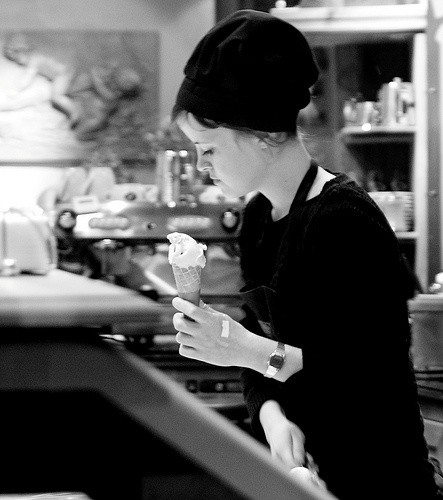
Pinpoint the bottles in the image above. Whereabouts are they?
[158,152,194,206]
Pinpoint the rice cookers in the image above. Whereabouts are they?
[0,212,58,276]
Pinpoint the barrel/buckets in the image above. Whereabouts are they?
[407,300,443,366]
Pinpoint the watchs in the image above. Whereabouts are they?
[264,342,284,379]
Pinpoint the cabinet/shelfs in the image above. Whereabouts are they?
[268,0,443,292]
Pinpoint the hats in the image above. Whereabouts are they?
[177,10,319,133]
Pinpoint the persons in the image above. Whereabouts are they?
[171,9,438,500]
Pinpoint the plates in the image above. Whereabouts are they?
[368,190,413,232]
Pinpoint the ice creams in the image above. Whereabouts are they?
[166,232,207,322]
